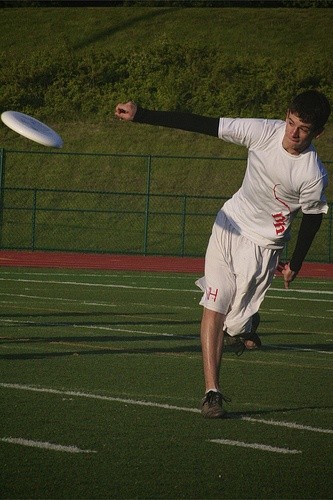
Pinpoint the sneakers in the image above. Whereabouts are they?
[240,333,261,350]
[202,389,226,418]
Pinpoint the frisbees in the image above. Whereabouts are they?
[1,111,64,149]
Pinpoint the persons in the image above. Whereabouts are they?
[114,88,330,420]
